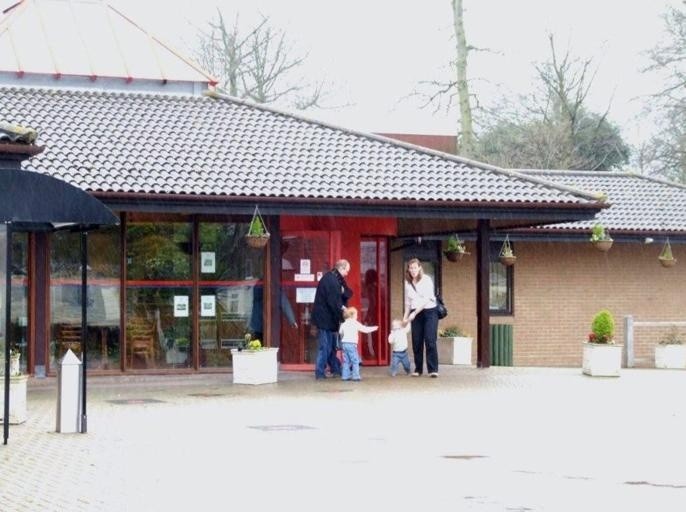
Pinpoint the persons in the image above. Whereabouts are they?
[249,277,298,348]
[309,259,353,380]
[402,258,439,377]
[338,306,379,382]
[387,319,412,378]
[363,267,385,360]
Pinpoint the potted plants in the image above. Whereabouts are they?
[590,223,614,252]
[499,241,517,265]
[442,236,471,262]
[0,349,22,376]
[581,310,623,376]
[657,246,674,267]
[244,216,271,249]
[436,324,473,365]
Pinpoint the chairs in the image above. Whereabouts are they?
[57,302,159,369]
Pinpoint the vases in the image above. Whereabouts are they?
[654,343,686,369]
[230,347,280,385]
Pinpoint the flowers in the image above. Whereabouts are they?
[234,334,262,352]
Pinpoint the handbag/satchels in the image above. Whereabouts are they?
[435,295,448,319]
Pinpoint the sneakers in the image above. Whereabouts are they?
[315,372,439,382]
